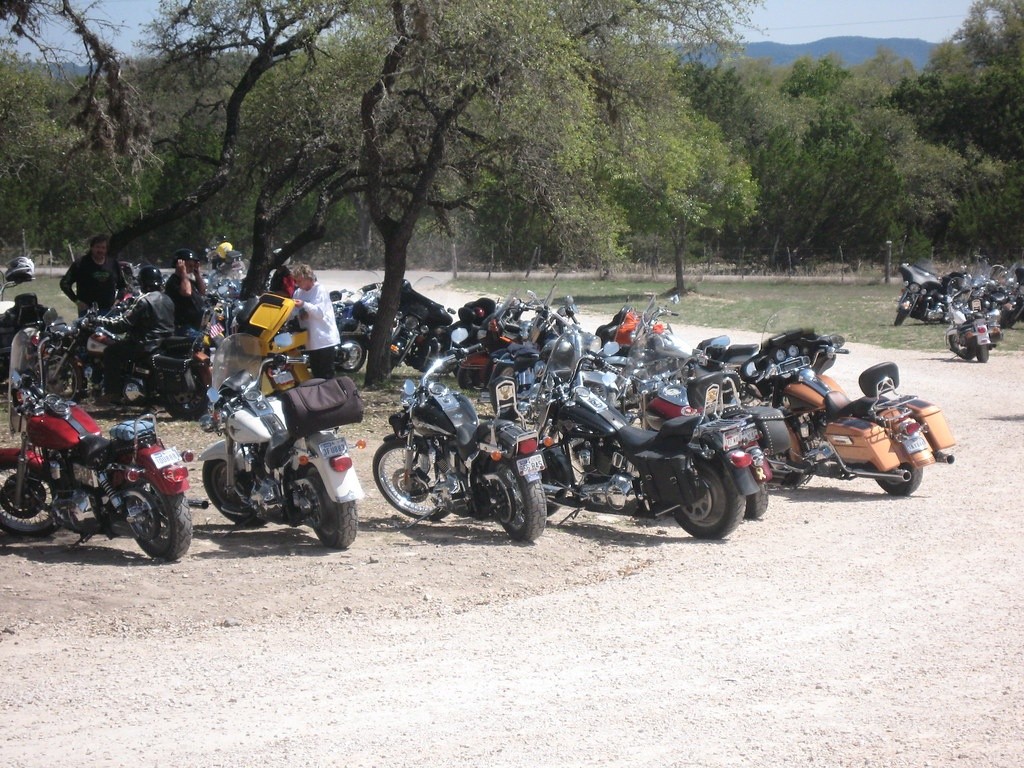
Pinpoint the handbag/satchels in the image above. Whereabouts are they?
[282,375,364,439]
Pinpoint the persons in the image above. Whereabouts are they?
[59,234,206,406]
[283,264,341,378]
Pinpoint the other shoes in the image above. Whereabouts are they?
[94,393,122,407]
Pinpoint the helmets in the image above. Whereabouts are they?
[172,249,198,269]
[215,242,233,259]
[137,266,163,290]
[6,256,35,284]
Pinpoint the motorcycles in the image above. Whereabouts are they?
[891,257,1024,363]
[198,333,365,548]
[740,308,957,496]
[1,306,209,561]
[371,341,549,540]
[517,329,759,539]
[0,265,792,520]
